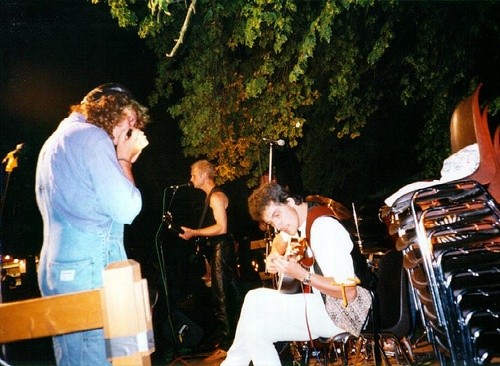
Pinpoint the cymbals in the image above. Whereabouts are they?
[305,195,353,220]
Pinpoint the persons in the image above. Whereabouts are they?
[36,83,150,366]
[178,160,240,362]
[219,181,379,366]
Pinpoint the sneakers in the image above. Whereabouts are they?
[203,347,227,362]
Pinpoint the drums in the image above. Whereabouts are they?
[250,238,274,280]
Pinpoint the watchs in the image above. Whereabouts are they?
[302,272,313,286]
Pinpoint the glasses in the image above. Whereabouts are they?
[95,83,135,98]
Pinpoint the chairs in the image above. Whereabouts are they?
[302,83,500,366]
[0,259,156,366]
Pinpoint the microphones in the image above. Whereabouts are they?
[3,143,27,162]
[263,138,285,146]
[170,182,192,188]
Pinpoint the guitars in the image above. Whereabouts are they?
[162,211,211,287]
[276,237,308,294]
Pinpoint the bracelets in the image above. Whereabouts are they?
[193,230,199,236]
[117,158,133,166]
